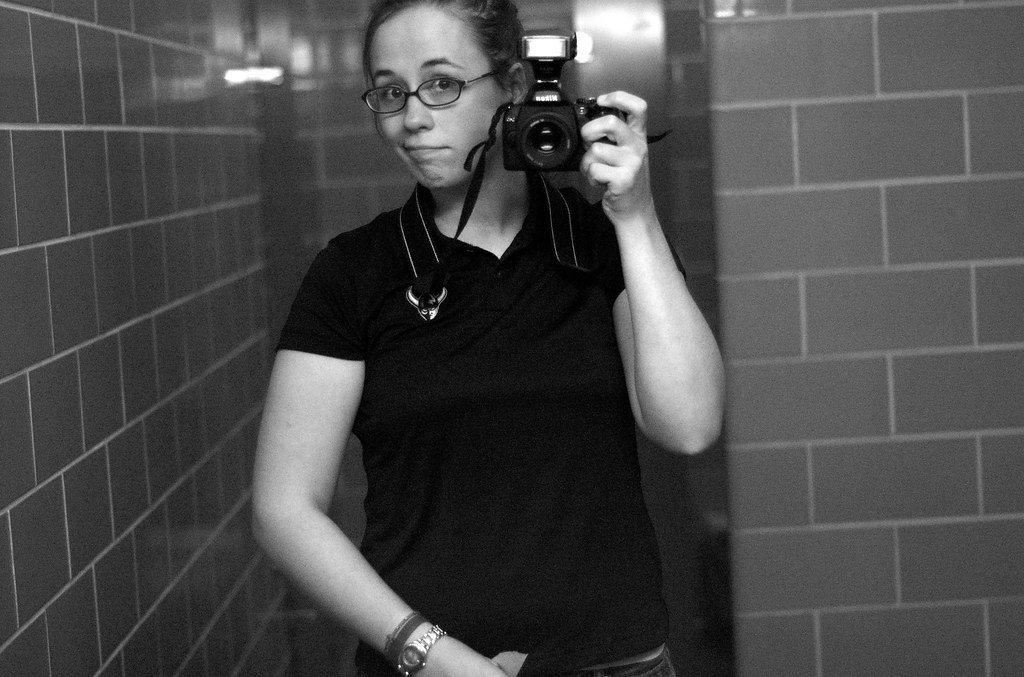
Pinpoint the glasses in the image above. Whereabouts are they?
[361,66,508,114]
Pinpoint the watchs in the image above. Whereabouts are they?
[396,624,448,676]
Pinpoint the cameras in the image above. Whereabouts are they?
[502,28,628,174]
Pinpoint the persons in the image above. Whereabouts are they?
[247,0,729,677]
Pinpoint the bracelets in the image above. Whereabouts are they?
[381,610,418,656]
[387,613,426,663]
